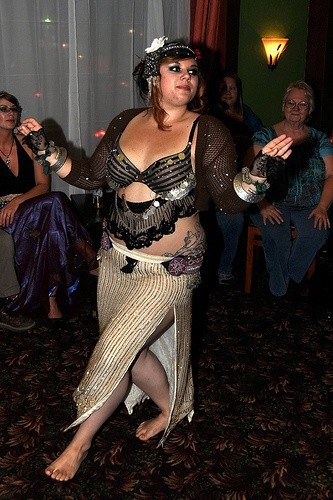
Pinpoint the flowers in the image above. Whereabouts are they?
[144,36,169,54]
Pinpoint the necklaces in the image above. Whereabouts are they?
[0,137,14,168]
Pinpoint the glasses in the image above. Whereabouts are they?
[282,99,311,112]
[0,106,24,114]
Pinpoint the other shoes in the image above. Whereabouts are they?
[0,307,37,331]
[217,270,235,286]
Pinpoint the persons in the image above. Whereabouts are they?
[202,70,265,285]
[245,80,333,308]
[0,229,35,332]
[0,91,99,337]
[17,38,293,482]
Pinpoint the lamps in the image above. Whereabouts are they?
[261,37,289,72]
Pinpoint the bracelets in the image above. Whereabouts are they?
[50,147,67,172]
[35,140,55,175]
[233,172,266,203]
[241,167,269,193]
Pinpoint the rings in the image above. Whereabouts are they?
[7,216,10,217]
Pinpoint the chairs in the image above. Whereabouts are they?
[245,219,317,295]
